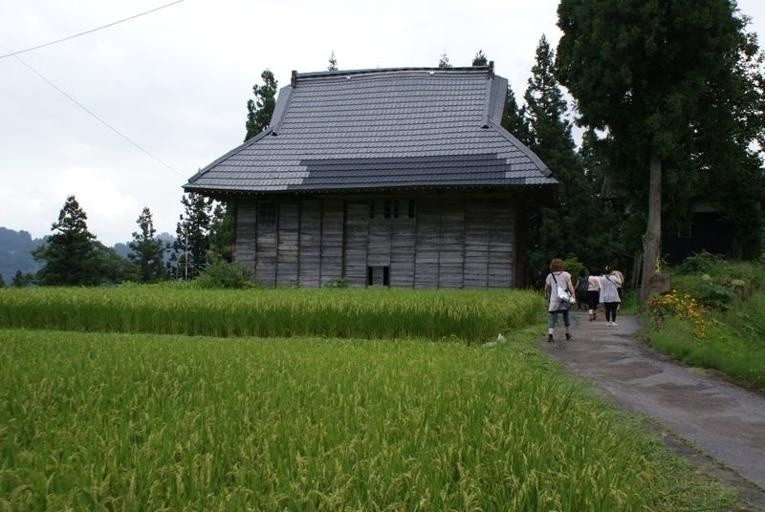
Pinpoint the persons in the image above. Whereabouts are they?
[584,267,601,321]
[598,265,622,327]
[574,270,588,311]
[610,262,625,312]
[545,258,576,342]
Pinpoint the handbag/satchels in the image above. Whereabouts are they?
[557,286,576,304]
